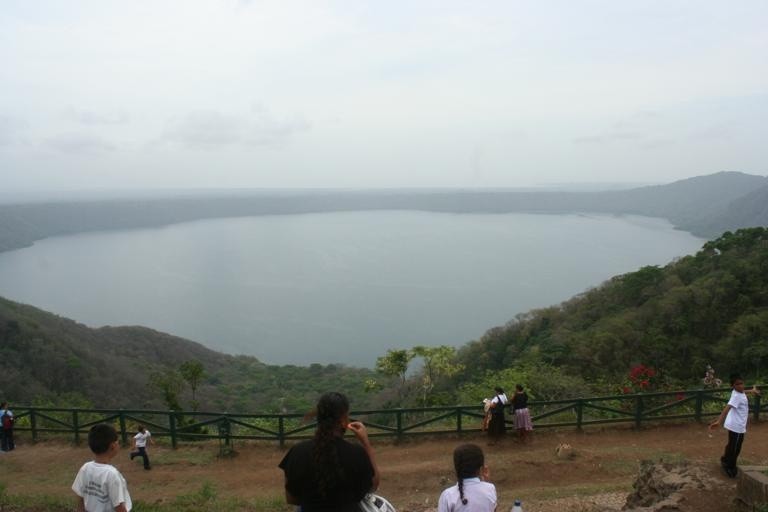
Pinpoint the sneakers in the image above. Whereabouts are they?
[487,440,531,445]
[721,458,738,478]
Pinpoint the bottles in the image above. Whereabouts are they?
[511,499,522,512]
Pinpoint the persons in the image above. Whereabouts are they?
[481,387,510,446]
[0,402,17,454]
[71,423,133,512]
[708,373,761,479]
[130,425,156,469]
[437,443,499,512]
[278,392,381,512]
[509,384,533,444]
